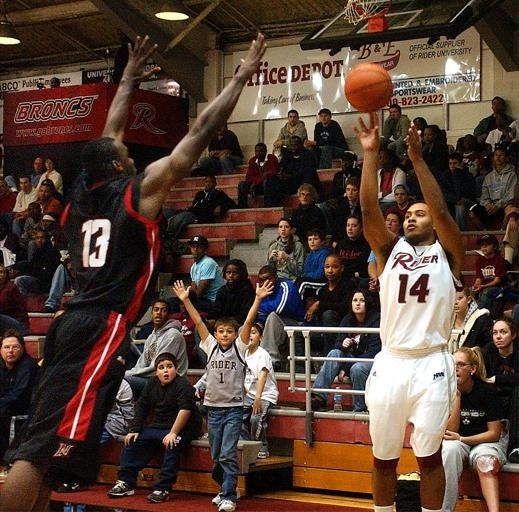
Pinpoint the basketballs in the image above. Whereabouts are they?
[344,62,393,111]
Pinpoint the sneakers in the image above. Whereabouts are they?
[63,289,79,297]
[505,260,513,270]
[41,306,55,313]
[217,499,237,512]
[300,399,328,412]
[508,448,519,463]
[212,487,240,505]
[258,451,269,459]
[109,479,135,496]
[198,433,208,440]
[147,490,170,503]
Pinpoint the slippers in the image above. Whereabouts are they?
[0,470,7,483]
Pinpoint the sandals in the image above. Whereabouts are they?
[58,482,79,491]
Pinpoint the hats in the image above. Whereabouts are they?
[477,234,497,245]
[394,184,409,193]
[187,236,208,248]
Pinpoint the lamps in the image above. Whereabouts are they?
[0,0,21,46]
[154,0,190,22]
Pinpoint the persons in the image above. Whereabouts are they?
[78,378,136,490]
[353,115,465,511]
[0,327,39,484]
[1,96,518,413]
[1,32,269,512]
[484,315,519,465]
[239,323,280,460]
[441,346,507,512]
[170,278,275,512]
[106,352,204,503]
[49,76,62,90]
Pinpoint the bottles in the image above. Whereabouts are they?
[332,387,343,413]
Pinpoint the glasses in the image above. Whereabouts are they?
[455,363,471,369]
[3,344,20,351]
